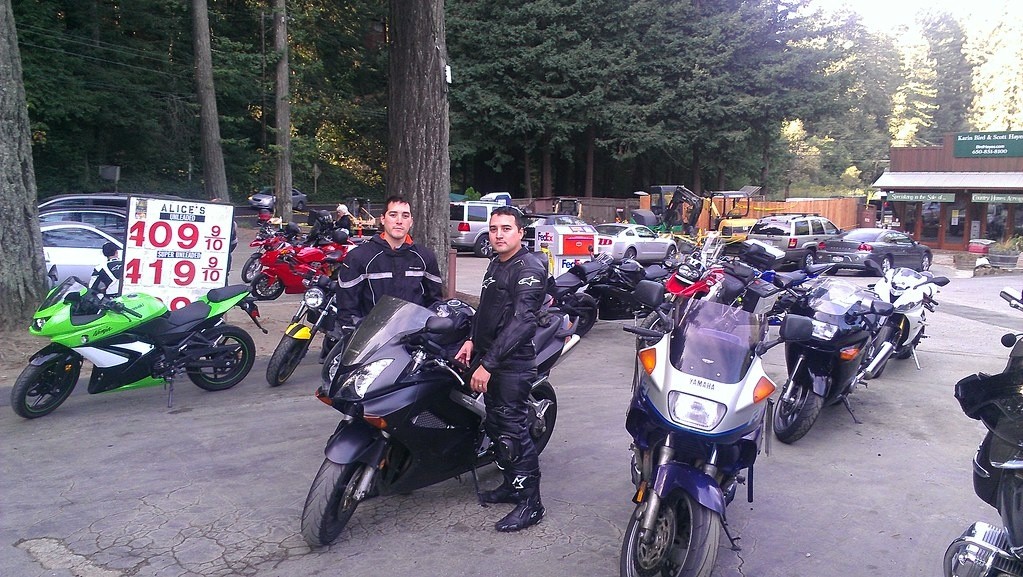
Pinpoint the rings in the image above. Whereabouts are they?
[479,384,483,388]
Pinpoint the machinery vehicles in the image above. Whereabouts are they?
[344,197,379,236]
[552,185,757,256]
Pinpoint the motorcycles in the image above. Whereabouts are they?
[620,295,813,577]
[860,257,950,380]
[265,254,344,387]
[300,294,580,547]
[942,289,1023,577]
[241,206,368,301]
[547,232,836,338]
[10,264,268,419]
[635,244,780,350]
[772,275,896,444]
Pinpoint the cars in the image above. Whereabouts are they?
[38,206,127,243]
[745,212,846,270]
[39,220,125,286]
[37,192,238,253]
[248,186,307,210]
[595,223,679,263]
[520,213,588,249]
[449,200,526,258]
[816,228,933,277]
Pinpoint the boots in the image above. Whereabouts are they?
[493,468,545,533]
[475,467,523,505]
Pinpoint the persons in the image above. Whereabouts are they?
[336,192,447,373]
[88,240,127,300]
[336,204,354,237]
[206,197,239,326]
[453,204,549,532]
